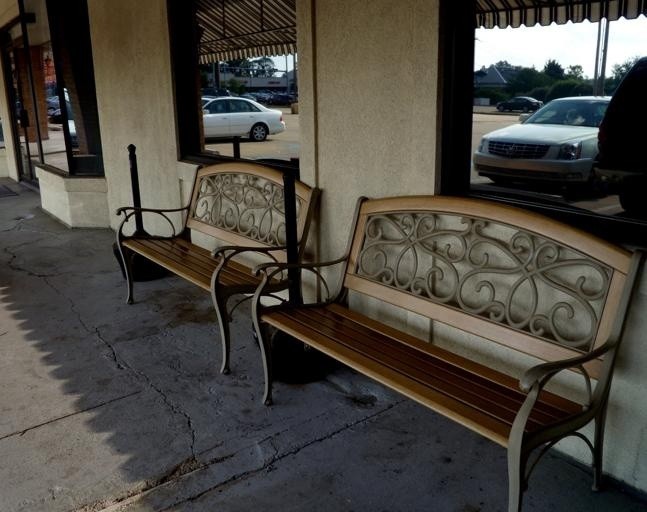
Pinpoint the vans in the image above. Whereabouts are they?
[589,54,646,240]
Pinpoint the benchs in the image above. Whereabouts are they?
[114,159,322,375]
[250,196,647,512]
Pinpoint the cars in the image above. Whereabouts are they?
[201,96,286,141]
[496,97,543,112]
[473,95,612,197]
[256,94,295,105]
